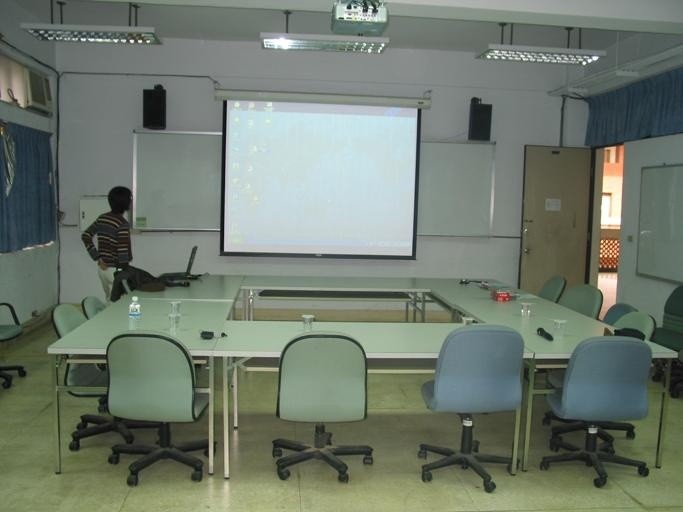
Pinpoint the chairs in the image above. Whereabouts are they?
[539,336,652,488]
[106,330,217,487]
[0,303,27,389]
[418,324,524,491]
[273,331,374,484]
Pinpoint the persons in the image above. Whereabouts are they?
[81,186,133,301]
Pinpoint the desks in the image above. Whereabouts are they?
[49,272,682,479]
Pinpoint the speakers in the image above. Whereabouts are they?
[143,89,166,130]
[469,103,492,141]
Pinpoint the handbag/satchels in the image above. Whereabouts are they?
[111,263,136,301]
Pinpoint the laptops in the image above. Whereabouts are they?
[159,245,197,280]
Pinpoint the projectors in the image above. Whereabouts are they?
[331,3,388,37]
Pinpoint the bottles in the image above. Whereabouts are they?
[127,296,142,320]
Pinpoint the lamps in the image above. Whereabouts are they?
[260,9,389,55]
[21,0,165,46]
[473,22,608,68]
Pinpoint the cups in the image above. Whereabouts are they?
[167,316,179,329]
[301,314,314,331]
[553,319,568,336]
[168,301,180,316]
[521,302,536,315]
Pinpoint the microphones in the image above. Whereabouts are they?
[537,327,553,341]
